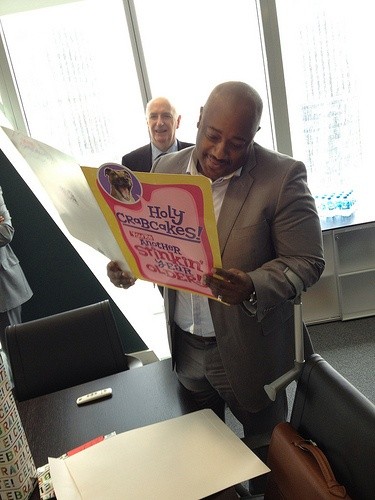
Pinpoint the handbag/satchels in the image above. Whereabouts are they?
[264,422,352,500]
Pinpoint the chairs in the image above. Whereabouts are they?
[3,300,130,402]
[234,354,375,500]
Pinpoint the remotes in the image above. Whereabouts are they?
[76,388,112,405]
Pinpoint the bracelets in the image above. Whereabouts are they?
[246,285,257,306]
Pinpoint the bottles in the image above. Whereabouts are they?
[314,190,357,217]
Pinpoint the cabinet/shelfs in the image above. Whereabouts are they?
[301,216,375,326]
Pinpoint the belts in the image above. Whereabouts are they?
[172,322,218,351]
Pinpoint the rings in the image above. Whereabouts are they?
[119,283,124,288]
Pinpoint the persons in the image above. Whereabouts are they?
[0,185,34,362]
[122,96,196,297]
[106,82,326,495]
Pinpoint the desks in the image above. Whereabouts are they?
[17,357,195,470]
